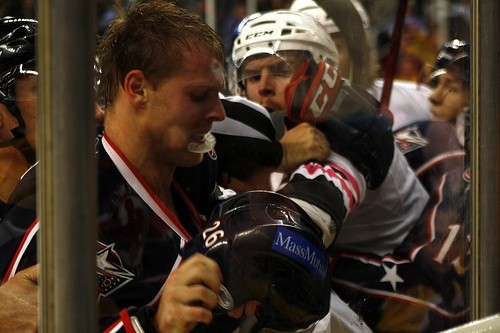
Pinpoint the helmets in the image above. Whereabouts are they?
[178,190,330,333]
[290,0,373,34]
[210,91,277,144]
[0,17,102,102]
[429,39,470,86]
[231,9,340,70]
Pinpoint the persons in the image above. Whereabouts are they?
[0,1,473,332]
